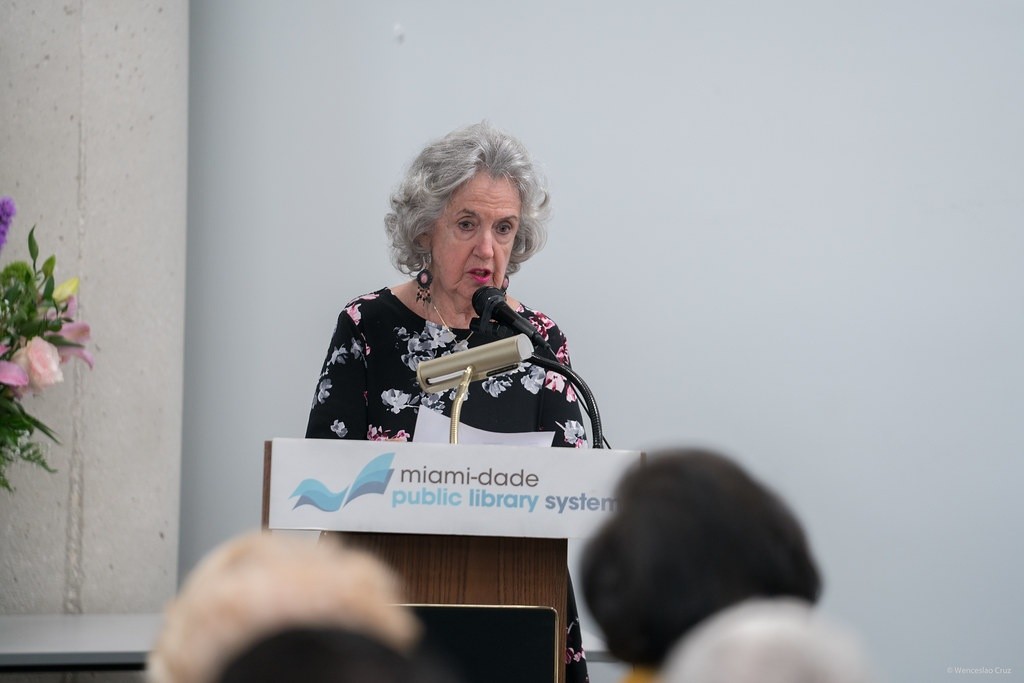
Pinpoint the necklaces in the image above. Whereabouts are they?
[434,306,473,344]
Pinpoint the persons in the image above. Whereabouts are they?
[147,526,454,683]
[577,447,907,683]
[306,119,589,683]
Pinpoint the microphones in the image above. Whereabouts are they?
[472,286,549,351]
